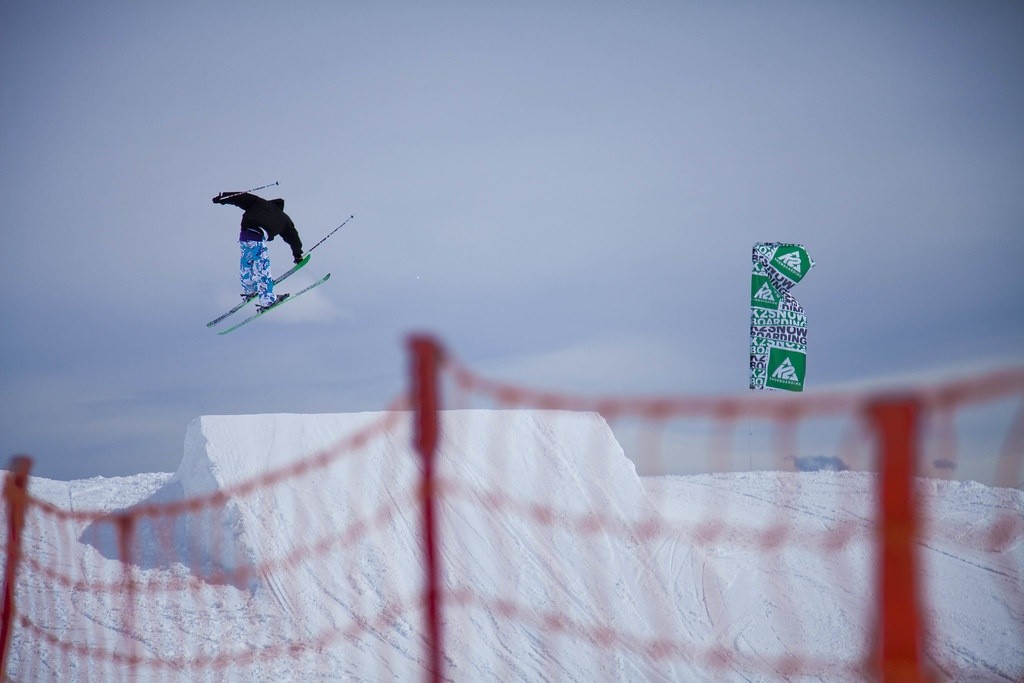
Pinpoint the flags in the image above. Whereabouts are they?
[747,241,816,395]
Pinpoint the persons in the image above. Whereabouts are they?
[212,190,303,310]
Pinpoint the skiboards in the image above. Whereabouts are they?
[206,254,331,336]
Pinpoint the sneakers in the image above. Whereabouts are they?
[240,293,258,302]
[257,293,287,314]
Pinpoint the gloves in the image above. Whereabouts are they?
[293,256,303,264]
[212,196,221,203]
